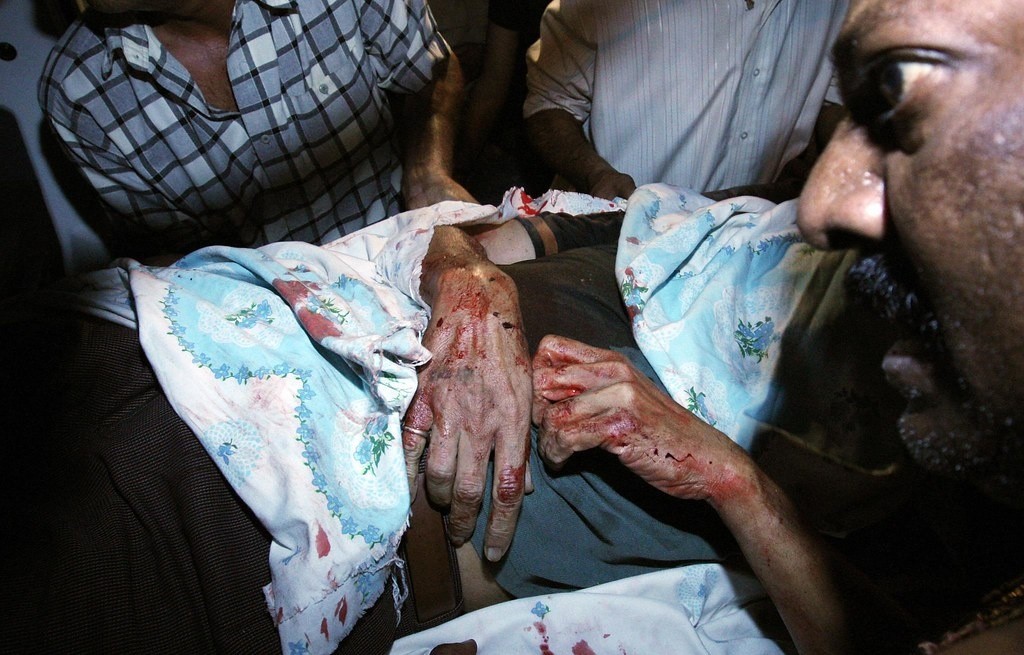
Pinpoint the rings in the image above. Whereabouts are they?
[403,425,428,438]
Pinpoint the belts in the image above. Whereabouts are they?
[401,468,457,624]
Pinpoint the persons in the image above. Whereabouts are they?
[36,0,486,265]
[0,190,918,655]
[524,0,849,203]
[796,0,1024,653]
